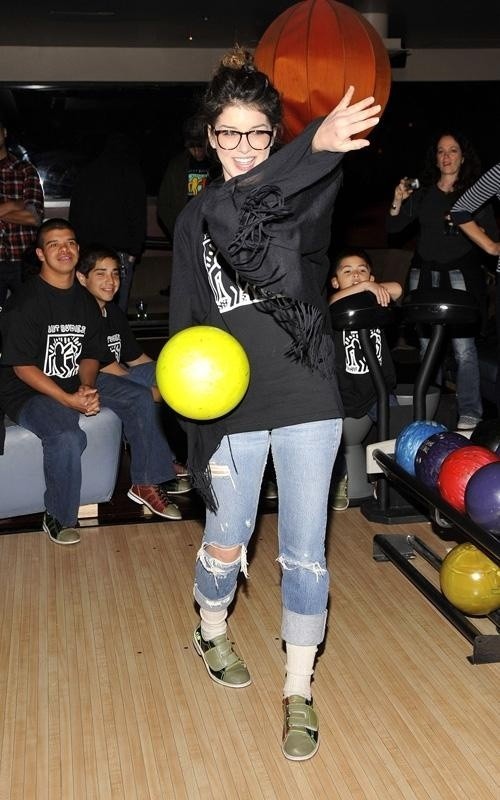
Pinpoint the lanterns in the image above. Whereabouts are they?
[253,0,391,145]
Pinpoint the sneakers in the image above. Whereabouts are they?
[192,621,253,688]
[159,477,195,493]
[456,414,481,430]
[173,461,191,477]
[281,684,319,760]
[127,483,183,520]
[262,477,278,498]
[41,509,81,545]
[327,475,350,512]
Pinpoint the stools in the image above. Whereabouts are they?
[1,405,123,528]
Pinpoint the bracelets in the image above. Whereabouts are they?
[390,203,396,210]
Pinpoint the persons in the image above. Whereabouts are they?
[449,163,500,287]
[75,245,193,494]
[68,134,147,312]
[385,132,500,432]
[0,218,183,545]
[156,116,221,241]
[0,122,45,306]
[171,54,385,759]
[328,250,403,510]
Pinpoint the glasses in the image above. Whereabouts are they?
[206,118,275,151]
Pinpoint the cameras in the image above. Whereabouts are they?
[404,178,419,190]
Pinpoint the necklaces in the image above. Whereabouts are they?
[438,181,453,189]
